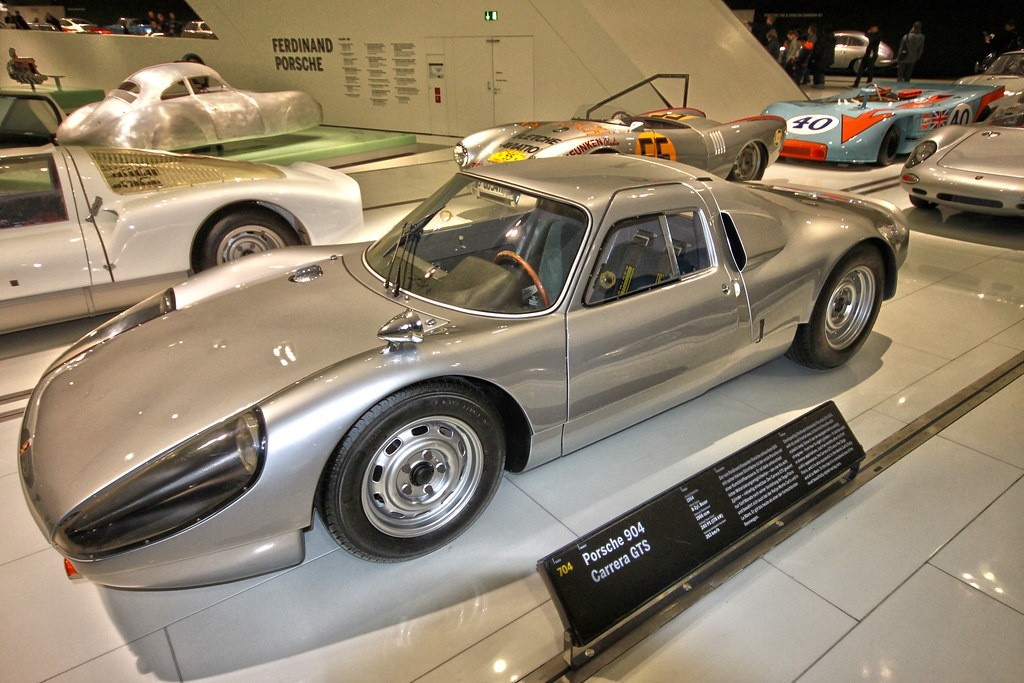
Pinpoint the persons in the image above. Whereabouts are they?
[847,24,882,90]
[149,10,182,36]
[4,9,32,30]
[743,14,835,89]
[896,22,925,83]
[45,11,62,31]
[988,17,1019,60]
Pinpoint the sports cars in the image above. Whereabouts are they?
[453,73,788,183]
[0,57,366,335]
[951,49,1024,96]
[899,90,1024,218]
[759,83,1006,166]
[15,151,911,591]
[777,30,897,77]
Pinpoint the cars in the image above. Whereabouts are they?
[1,4,221,38]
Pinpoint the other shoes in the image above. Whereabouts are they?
[848,85,859,89]
[811,84,825,89]
[800,82,806,86]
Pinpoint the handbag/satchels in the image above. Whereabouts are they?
[898,35,908,60]
